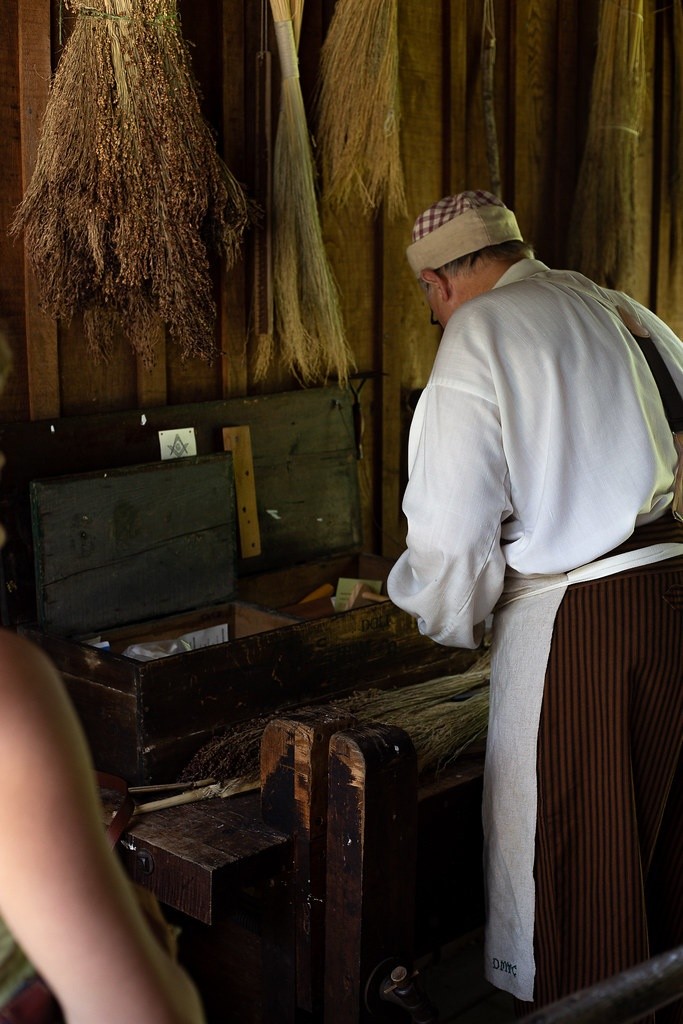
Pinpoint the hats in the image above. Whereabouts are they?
[405,190,525,277]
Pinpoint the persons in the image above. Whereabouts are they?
[386,188,683,1024]
[1,627,205,1024]
